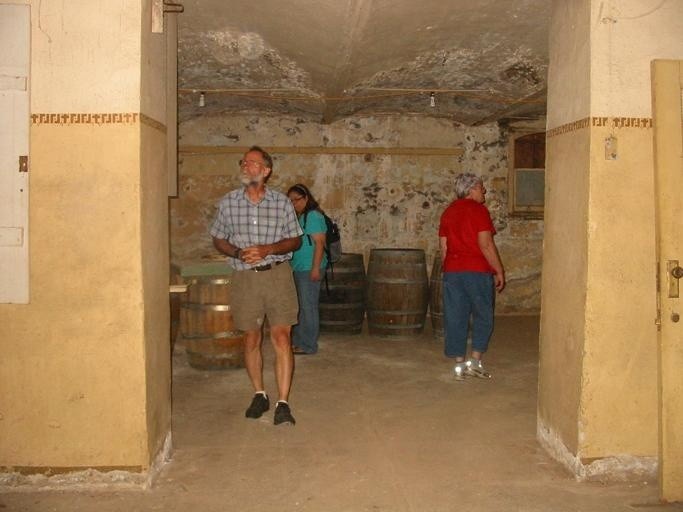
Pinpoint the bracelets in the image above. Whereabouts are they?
[234,248,241,259]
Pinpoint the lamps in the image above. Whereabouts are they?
[199,93,204,106]
[430,92,435,107]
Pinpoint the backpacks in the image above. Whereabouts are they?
[303,209,342,264]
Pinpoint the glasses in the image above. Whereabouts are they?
[240,159,266,168]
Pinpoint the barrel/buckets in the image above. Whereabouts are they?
[170,266,180,353]
[429,251,473,344]
[180,275,244,370]
[365,249,429,339]
[318,253,366,336]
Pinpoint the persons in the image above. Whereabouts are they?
[285,182,327,355]
[438,173,504,382]
[209,143,305,429]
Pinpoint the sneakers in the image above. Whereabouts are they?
[448,357,466,382]
[464,359,492,380]
[246,394,269,418]
[289,343,313,355]
[273,404,296,428]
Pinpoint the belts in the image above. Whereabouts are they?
[247,260,284,273]
